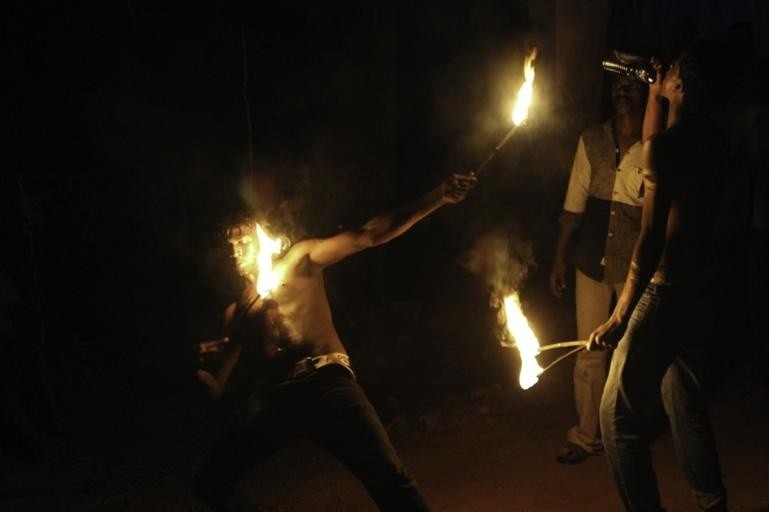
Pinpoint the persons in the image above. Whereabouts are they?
[188,171,478,512]
[547,52,652,463]
[584,55,728,512]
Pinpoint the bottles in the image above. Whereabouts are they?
[600,46,664,85]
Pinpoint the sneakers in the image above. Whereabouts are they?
[556,441,588,463]
